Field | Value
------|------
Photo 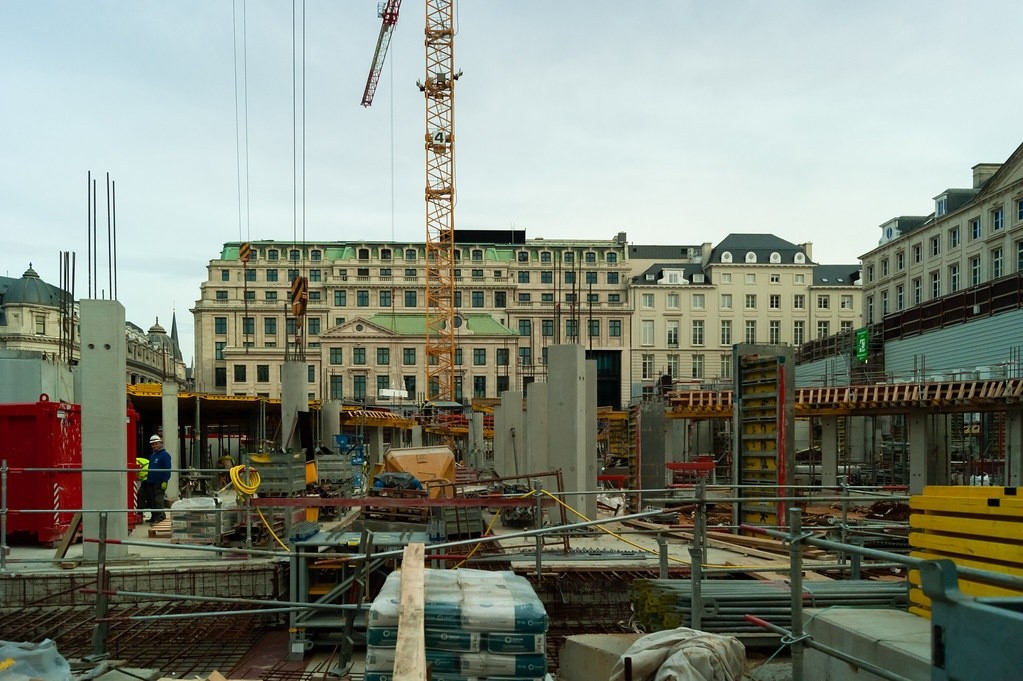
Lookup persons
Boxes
[145,435,172,523]
[218,448,235,489]
[135,457,151,517]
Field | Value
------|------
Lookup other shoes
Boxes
[146,514,166,523]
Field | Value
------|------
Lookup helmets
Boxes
[149,434,163,444]
[223,449,228,453]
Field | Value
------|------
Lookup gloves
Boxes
[161,482,167,489]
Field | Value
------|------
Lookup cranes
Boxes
[359,0,463,511]
